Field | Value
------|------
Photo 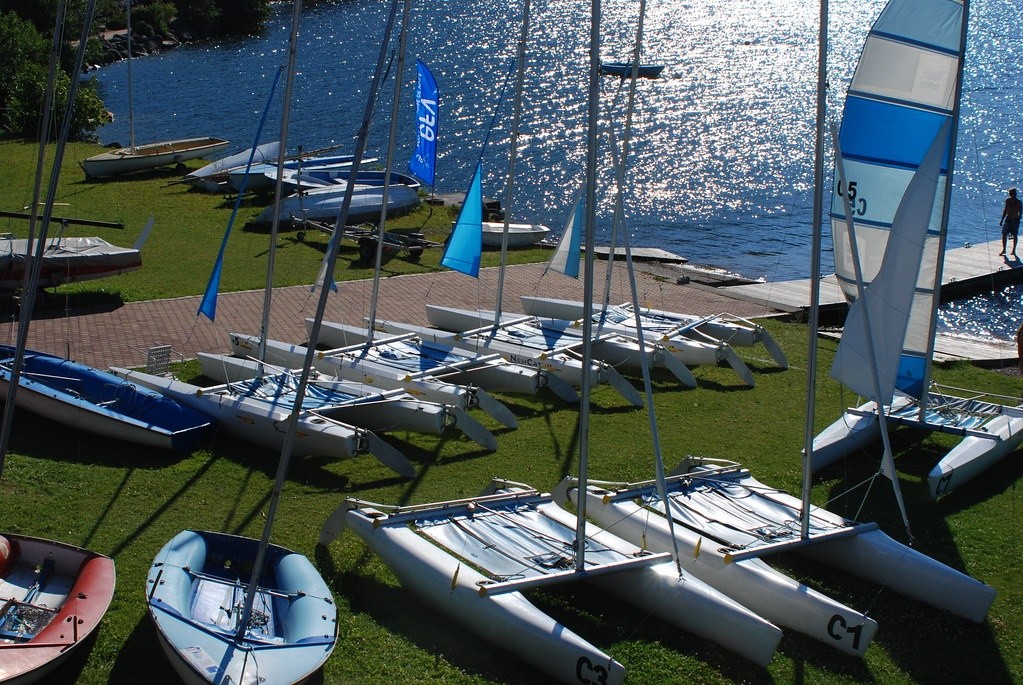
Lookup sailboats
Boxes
[0,0,998,685]
[799,0,1022,505]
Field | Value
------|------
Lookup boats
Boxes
[600,62,664,79]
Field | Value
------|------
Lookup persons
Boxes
[1016,323,1023,372]
[999,187,1023,256]
